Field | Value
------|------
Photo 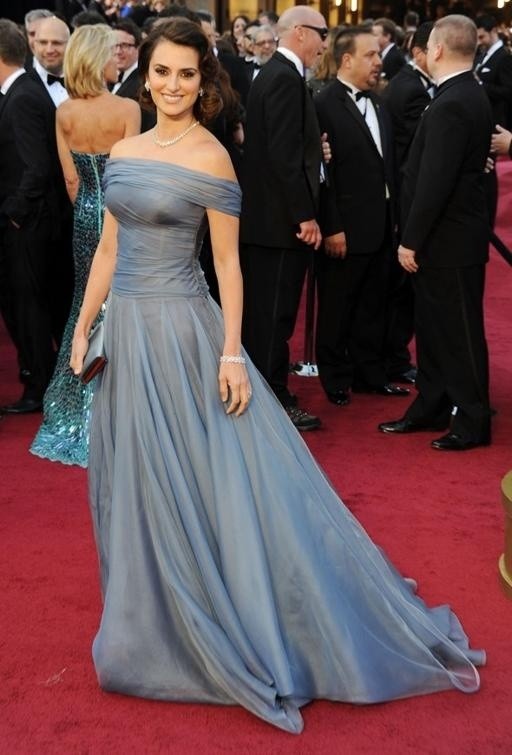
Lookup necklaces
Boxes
[151,121,202,147]
[53,17,486,738]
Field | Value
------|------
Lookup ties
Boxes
[479,51,487,64]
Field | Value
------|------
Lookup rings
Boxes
[247,389,253,399]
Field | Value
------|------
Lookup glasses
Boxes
[243,34,251,39]
[116,42,136,48]
[254,40,275,46]
[304,25,330,41]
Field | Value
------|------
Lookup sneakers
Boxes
[286,405,321,431]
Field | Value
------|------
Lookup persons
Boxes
[29,17,77,107]
[489,125,512,156]
[110,23,144,105]
[75,13,108,28]
[228,15,244,42]
[0,18,74,414]
[233,27,277,99]
[193,12,218,49]
[30,24,141,469]
[78,0,202,23]
[22,10,47,59]
[374,12,495,451]
[231,21,257,63]
[235,7,323,431]
[258,12,276,28]
[312,29,411,407]
[370,18,407,76]
[382,16,443,164]
[472,16,511,121]
[314,21,348,80]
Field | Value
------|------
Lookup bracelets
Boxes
[219,355,246,365]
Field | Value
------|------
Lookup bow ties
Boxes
[419,69,435,91]
[46,73,65,86]
[347,88,374,102]
[252,62,263,71]
[118,71,124,82]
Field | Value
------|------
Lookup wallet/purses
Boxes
[79,321,108,386]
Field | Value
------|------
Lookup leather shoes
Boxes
[329,388,351,406]
[433,432,485,451]
[403,368,418,383]
[0,391,44,414]
[379,384,411,395]
[377,415,423,436]
